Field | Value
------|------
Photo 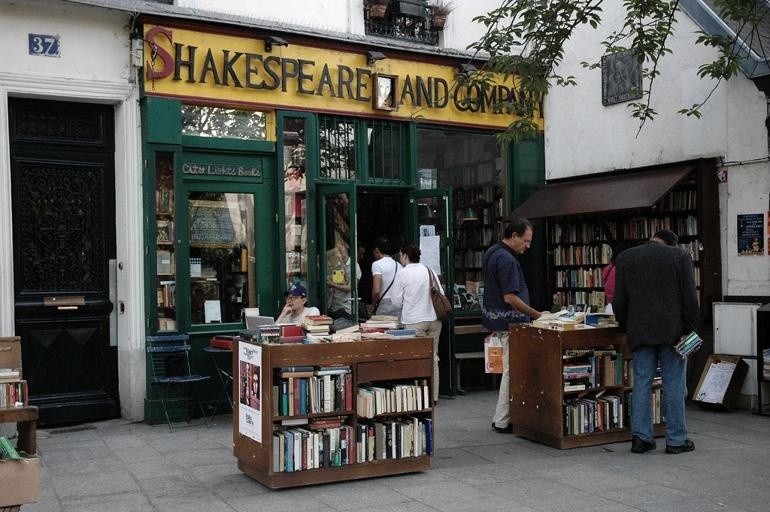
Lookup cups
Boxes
[675,331,703,358]
[561,306,566,312]
[568,305,574,318]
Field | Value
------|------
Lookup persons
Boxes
[602,255,617,308]
[393,245,454,406]
[372,237,405,321]
[613,230,697,454]
[482,218,552,433]
[274,285,319,326]
[326,242,362,334]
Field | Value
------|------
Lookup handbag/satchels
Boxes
[426,265,454,321]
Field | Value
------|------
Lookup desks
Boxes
[202,346,232,420]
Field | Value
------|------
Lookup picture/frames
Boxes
[372,71,399,112]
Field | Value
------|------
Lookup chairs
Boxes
[145,333,211,434]
[452,315,505,395]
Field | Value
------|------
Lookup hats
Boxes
[284,284,307,297]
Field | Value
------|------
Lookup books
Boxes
[156,220,174,244]
[555,270,605,287]
[156,186,174,213]
[656,388,661,425]
[357,418,434,463]
[652,394,656,424]
[274,367,353,415]
[441,144,497,167]
[655,188,698,209]
[454,223,503,245]
[273,416,353,472]
[454,208,498,223]
[158,281,175,308]
[554,244,614,263]
[529,310,618,331]
[553,215,697,242]
[453,271,485,308]
[674,243,701,260]
[451,185,500,206]
[246,308,273,332]
[654,367,665,390]
[556,291,606,308]
[562,348,634,388]
[190,261,218,279]
[0,383,22,405]
[159,313,174,331]
[188,197,246,242]
[356,380,430,418]
[241,315,417,345]
[439,163,500,183]
[565,394,628,435]
[156,250,176,275]
[454,246,485,268]
[762,349,770,378]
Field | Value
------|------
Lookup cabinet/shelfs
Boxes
[503,167,722,344]
[380,127,503,291]
[156,156,307,331]
[507,324,677,450]
[229,336,433,490]
[0,334,40,512]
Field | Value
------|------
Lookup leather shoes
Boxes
[631,435,657,454]
[665,439,695,454]
[492,422,495,430]
[495,422,513,433]
